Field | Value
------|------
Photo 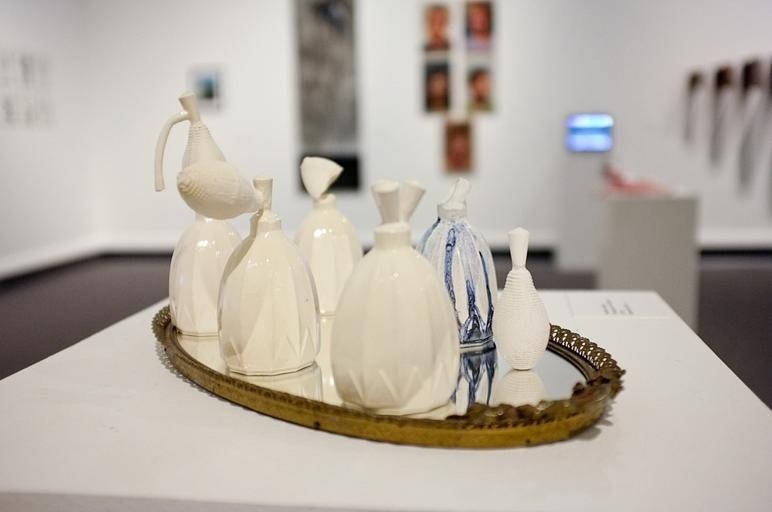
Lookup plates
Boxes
[152,306,627,450]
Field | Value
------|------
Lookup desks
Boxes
[0,288,770,510]
